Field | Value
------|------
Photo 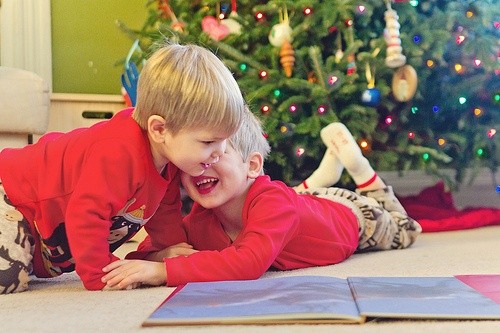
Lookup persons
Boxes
[105,100,424,288]
[0,44,246,295]
